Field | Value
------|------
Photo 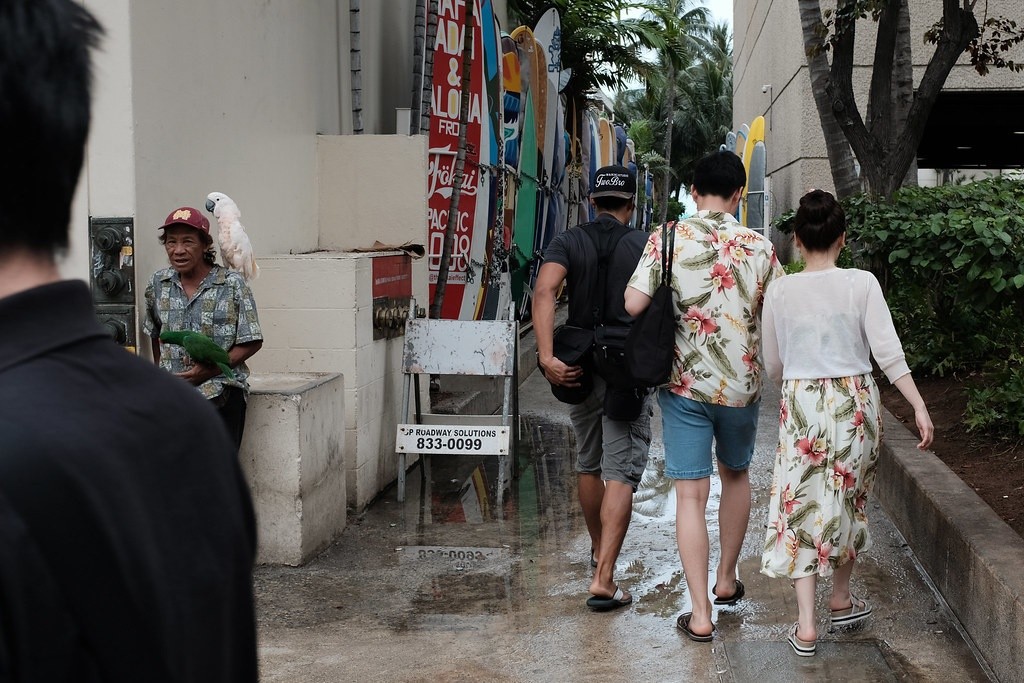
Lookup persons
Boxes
[0,0,265,683]
[762,190,933,656]
[623,151,788,642]
[141,207,265,456]
[531,163,650,610]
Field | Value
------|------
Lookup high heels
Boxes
[786,620,816,657]
[830,591,873,626]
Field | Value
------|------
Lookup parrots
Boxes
[204,192,260,283]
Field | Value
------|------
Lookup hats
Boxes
[590,164,636,200]
[158,207,210,235]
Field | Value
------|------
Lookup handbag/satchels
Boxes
[592,317,633,383]
[535,324,597,405]
[601,377,645,424]
[621,219,679,386]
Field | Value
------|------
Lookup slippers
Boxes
[712,579,745,605]
[591,547,599,567]
[677,612,716,642]
[586,586,633,608]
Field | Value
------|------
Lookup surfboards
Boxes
[425,0,655,321]
[718,116,767,236]
